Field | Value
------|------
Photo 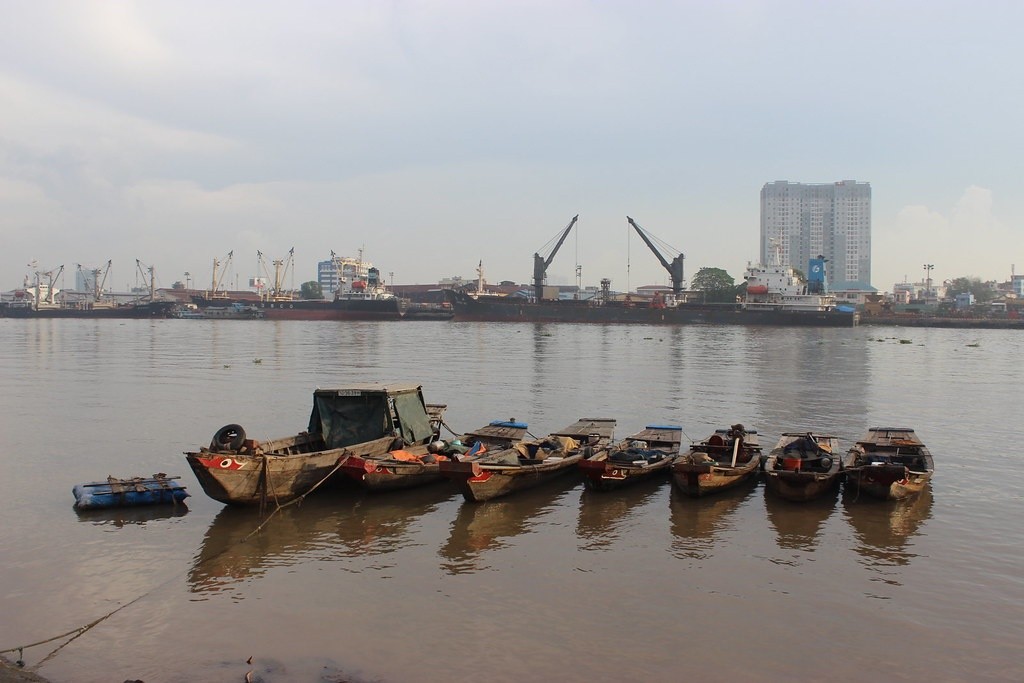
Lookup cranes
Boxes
[79,261,117,315]
[256,249,296,304]
[135,260,182,317]
[210,252,236,304]
[34,264,65,307]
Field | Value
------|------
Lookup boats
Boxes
[183,389,445,500]
[331,248,455,317]
[764,435,841,502]
[445,421,616,500]
[448,260,513,304]
[341,415,525,496]
[844,428,932,505]
[671,428,758,496]
[573,427,675,490]
[743,238,863,327]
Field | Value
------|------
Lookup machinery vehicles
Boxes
[530,215,583,309]
[627,213,686,307]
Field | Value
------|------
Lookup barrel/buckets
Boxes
[783,458,801,471]
